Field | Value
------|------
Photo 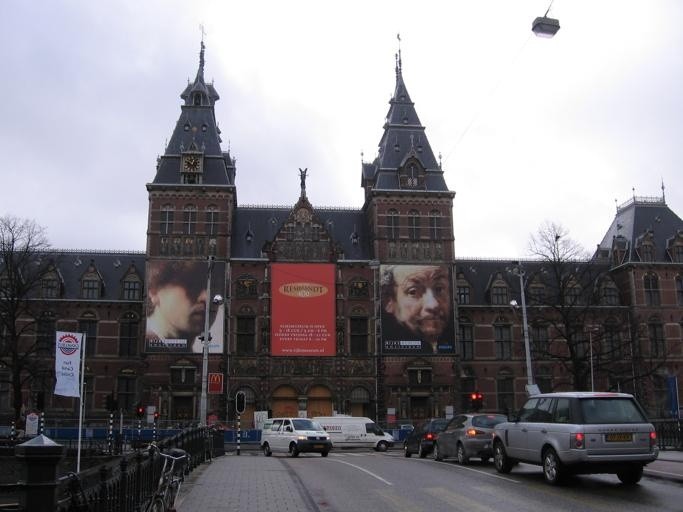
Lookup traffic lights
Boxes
[101,392,111,410]
[477,393,483,409]
[154,412,160,423]
[236,391,247,415]
[138,407,145,417]
[471,392,477,408]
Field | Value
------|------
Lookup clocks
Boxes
[178,151,204,175]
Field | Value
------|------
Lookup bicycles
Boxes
[198,424,223,463]
[138,440,190,512]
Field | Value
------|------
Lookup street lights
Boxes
[509,270,534,385]
[589,326,599,393]
[198,255,224,430]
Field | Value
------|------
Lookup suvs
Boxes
[492,390,661,487]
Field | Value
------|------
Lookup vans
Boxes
[261,417,333,458]
[313,416,395,452]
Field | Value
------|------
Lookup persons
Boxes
[380,264,455,354]
[146,259,224,352]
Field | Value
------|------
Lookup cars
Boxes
[404,416,452,459]
[433,412,509,465]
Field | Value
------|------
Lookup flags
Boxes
[54,331,83,398]
